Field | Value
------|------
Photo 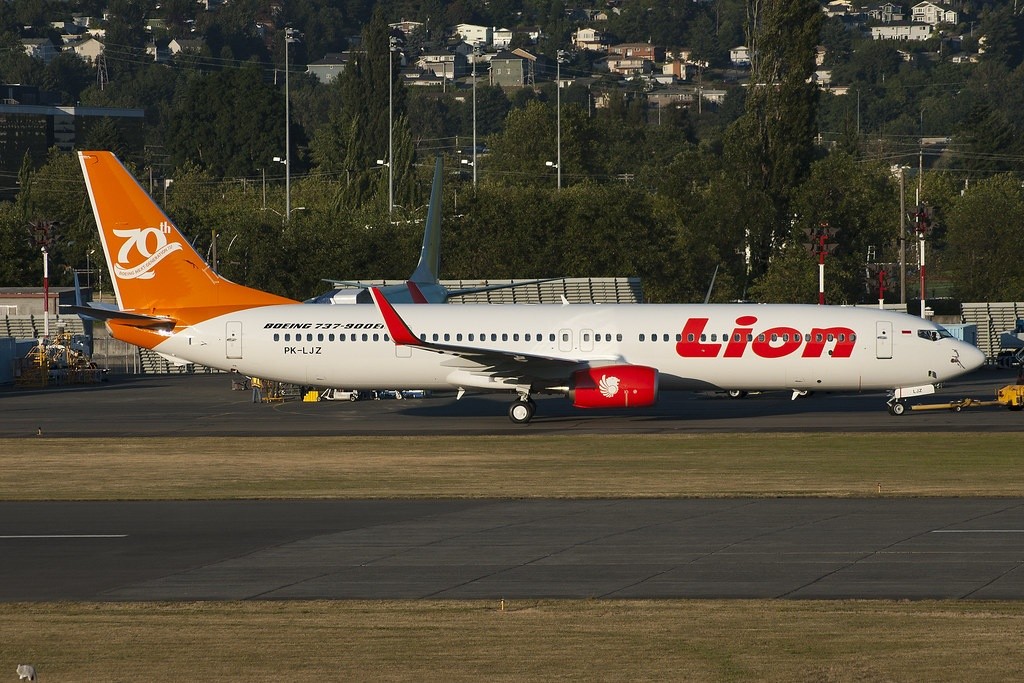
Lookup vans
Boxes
[322,385,372,401]
[384,385,430,398]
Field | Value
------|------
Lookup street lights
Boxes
[549,48,571,194]
[385,35,407,217]
[282,25,307,223]
[471,41,487,204]
[258,203,306,246]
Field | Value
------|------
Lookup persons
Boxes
[250,376,263,403]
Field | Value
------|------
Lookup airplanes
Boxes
[73,149,987,427]
[303,153,575,305]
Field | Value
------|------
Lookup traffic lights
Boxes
[906,207,919,237]
